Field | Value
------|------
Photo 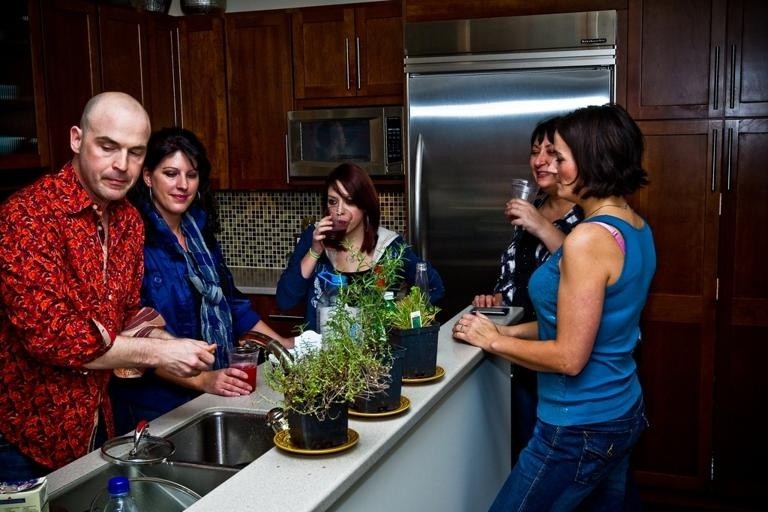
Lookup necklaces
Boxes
[584,203,631,218]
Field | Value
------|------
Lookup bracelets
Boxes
[310,247,319,261]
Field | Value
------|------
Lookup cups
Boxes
[226,344,261,394]
[319,206,354,249]
[503,177,539,226]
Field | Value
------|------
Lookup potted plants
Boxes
[384,289,439,379]
[264,321,391,449]
[325,237,413,412]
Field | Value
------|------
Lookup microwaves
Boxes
[285,105,405,181]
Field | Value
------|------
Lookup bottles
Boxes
[104,476,138,512]
[384,292,397,312]
[413,262,429,301]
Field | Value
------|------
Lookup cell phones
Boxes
[471,306,509,316]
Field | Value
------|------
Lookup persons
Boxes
[452,105,657,512]
[0,92,216,485]
[471,116,586,472]
[107,126,295,439]
[274,164,445,333]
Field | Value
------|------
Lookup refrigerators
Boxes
[402,9,621,324]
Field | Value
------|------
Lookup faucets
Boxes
[97,417,181,490]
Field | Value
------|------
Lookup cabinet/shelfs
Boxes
[0,0,230,194]
[225,8,294,191]
[287,0,405,189]
[626,0,768,512]
[28,306,522,512]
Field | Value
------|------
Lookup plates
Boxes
[274,427,359,456]
[402,366,447,386]
[348,393,410,419]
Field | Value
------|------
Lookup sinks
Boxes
[42,363,293,508]
